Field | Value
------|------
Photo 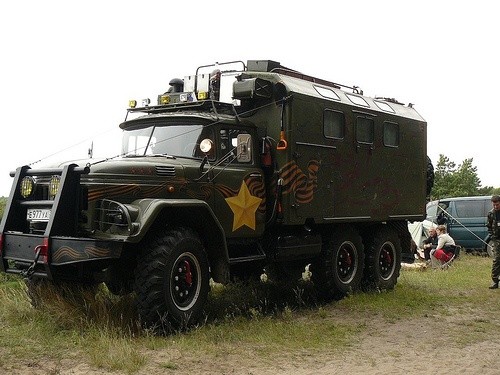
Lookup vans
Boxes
[425,195,494,258]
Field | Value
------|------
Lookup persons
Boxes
[430,225,455,261]
[486,195,500,289]
[423,227,438,262]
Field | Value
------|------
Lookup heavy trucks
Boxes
[1,58,434,338]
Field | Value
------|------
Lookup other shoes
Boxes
[489,283,498,289]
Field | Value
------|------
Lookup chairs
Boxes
[440,246,461,270]
